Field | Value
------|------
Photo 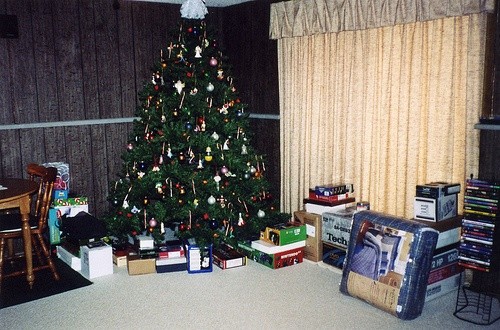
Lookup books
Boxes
[457,179,500,273]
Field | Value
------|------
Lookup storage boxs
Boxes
[42,162,460,301]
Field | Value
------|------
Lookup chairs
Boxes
[0,164,60,284]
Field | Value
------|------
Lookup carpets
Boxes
[0,212,92,311]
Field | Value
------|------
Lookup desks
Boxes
[0,178,38,289]
